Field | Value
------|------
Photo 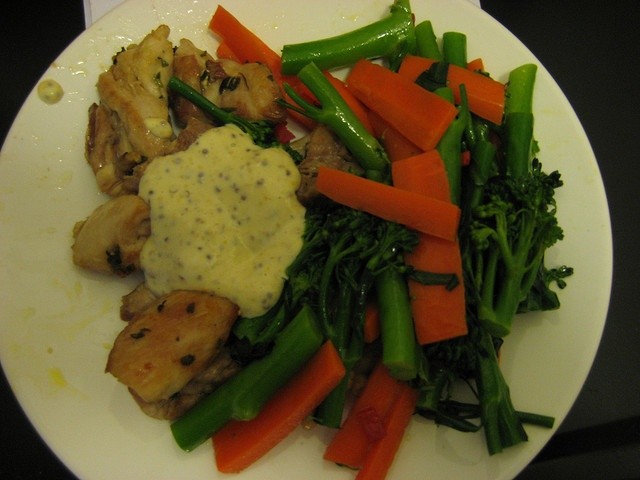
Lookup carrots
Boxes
[212,0,506,479]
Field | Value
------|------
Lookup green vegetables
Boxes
[165,1,574,454]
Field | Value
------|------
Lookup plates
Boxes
[0,0,614,480]
